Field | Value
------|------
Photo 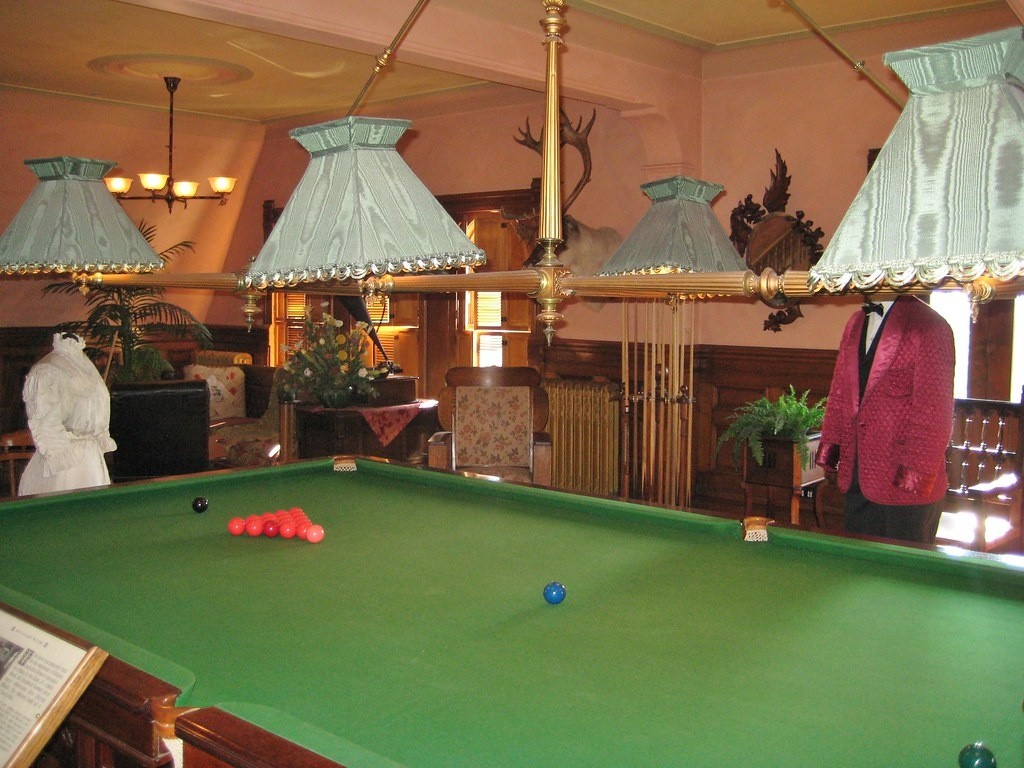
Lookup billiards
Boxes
[227,518,245,536]
[542,581,568,604]
[246,506,311,540]
[192,497,209,513]
[307,525,325,543]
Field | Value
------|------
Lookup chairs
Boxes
[2,431,33,495]
[427,365,552,487]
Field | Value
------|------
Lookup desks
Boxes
[0,451,1024,768]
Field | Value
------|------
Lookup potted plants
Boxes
[714,385,828,472]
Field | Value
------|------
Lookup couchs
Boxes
[108,366,279,482]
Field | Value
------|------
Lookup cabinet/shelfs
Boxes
[294,397,440,462]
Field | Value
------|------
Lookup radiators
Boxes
[538,381,626,495]
[197,349,253,365]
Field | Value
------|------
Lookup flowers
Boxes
[270,301,390,399]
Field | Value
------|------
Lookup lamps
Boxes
[597,177,752,273]
[240,115,491,292]
[0,155,165,274]
[805,27,1024,288]
[104,77,238,215]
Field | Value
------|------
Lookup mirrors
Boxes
[749,213,815,308]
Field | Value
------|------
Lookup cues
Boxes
[618,298,697,510]
[102,328,118,384]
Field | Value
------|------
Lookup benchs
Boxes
[812,398,1024,555]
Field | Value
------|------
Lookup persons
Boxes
[815,294,956,542]
[18,332,117,496]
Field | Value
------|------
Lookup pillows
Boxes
[183,364,247,421]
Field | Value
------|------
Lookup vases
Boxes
[319,388,352,407]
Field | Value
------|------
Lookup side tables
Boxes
[738,432,825,524]
